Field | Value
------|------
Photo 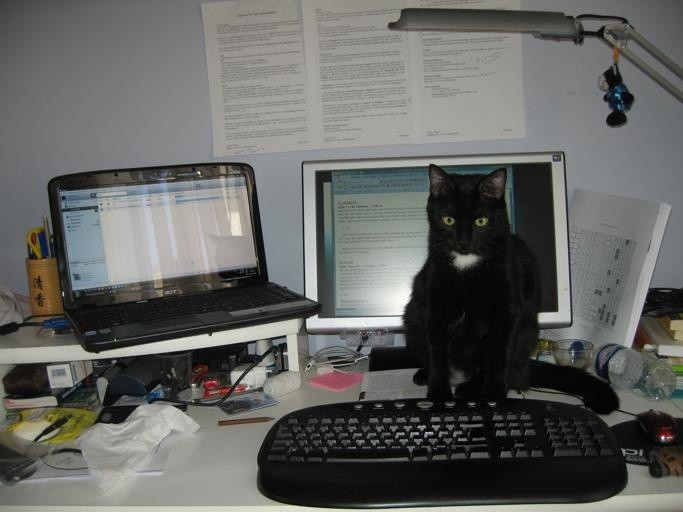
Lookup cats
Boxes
[401,163,620,416]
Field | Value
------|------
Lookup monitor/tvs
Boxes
[302,151,573,371]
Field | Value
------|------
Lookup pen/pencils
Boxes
[219,417,274,425]
[27,216,55,259]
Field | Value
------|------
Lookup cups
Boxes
[154,351,194,402]
[23,257,64,322]
[552,337,595,371]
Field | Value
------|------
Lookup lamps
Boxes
[384,8,681,106]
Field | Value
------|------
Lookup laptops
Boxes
[48,162,322,352]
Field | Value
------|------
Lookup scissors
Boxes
[27,226,45,259]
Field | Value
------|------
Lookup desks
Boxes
[2,353,683,510]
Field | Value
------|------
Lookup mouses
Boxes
[636,409,680,444]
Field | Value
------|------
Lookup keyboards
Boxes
[257,398,628,508]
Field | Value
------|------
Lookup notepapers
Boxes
[309,372,364,393]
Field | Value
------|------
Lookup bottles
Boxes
[263,370,303,397]
[590,342,676,399]
[254,340,276,366]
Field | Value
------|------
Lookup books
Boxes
[633,312,683,400]
